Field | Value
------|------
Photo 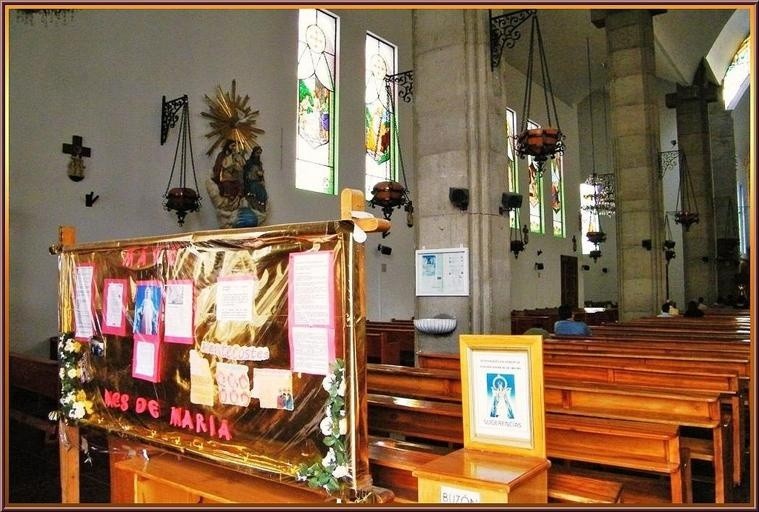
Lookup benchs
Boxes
[10,299,751,505]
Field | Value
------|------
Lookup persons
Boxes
[553,305,592,336]
[658,297,724,318]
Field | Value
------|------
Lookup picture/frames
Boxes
[414,248,470,296]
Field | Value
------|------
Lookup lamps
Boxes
[718,198,740,257]
[587,183,607,246]
[588,242,603,262]
[509,208,525,259]
[663,214,675,249]
[673,147,700,230]
[366,75,409,218]
[160,95,202,225]
[515,11,565,162]
